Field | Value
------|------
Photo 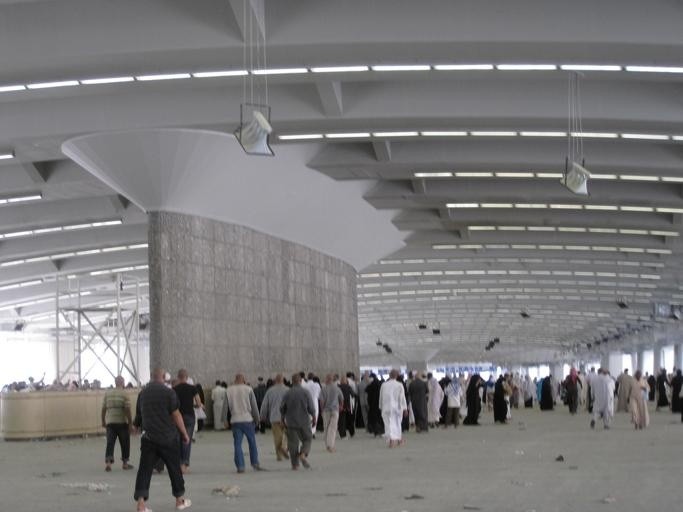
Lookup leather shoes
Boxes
[237,470,244,473]
[252,464,262,470]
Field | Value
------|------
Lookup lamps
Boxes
[230,0,280,158]
[555,70,597,199]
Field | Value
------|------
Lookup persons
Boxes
[132,364,191,512]
[485,365,683,431]
[2,371,100,391]
[163,368,484,475]
[100,374,134,472]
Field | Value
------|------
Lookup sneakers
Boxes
[176,499,191,510]
[276,447,310,470]
[144,507,153,512]
[106,466,111,472]
[123,464,134,469]
